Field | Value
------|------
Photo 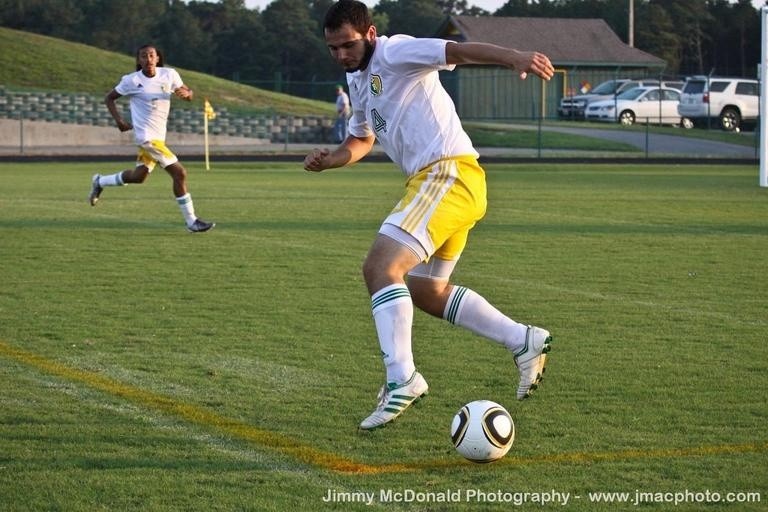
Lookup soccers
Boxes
[451,400,515,463]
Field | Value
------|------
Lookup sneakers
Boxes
[360,370,429,430]
[185,219,215,234]
[514,325,552,403]
[89,174,103,207]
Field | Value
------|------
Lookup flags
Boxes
[205,101,218,120]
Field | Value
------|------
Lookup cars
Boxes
[556,74,760,130]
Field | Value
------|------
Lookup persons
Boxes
[88,44,214,234]
[334,85,352,144]
[301,0,555,429]
